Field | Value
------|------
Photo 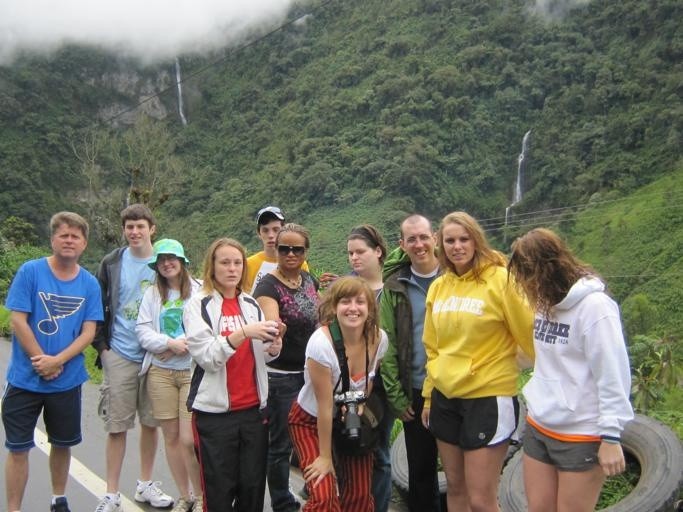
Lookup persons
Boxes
[422,213,535,510]
[182,239,282,511]
[377,214,447,511]
[504,227,636,511]
[91,204,175,512]
[344,227,393,308]
[1,211,105,511]
[135,238,204,512]
[288,275,389,511]
[240,206,312,297]
[250,224,327,511]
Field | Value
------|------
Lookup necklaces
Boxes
[275,265,304,289]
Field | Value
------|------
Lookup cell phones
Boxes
[263,321,277,349]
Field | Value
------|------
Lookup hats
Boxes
[148,239,189,270]
[256,206,284,224]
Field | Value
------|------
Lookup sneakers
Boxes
[51,496,70,512]
[135,480,174,507]
[171,495,203,512]
[95,493,123,512]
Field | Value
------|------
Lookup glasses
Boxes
[278,245,305,256]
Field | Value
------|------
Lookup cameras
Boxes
[333,390,368,440]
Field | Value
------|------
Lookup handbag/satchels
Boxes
[334,414,374,455]
